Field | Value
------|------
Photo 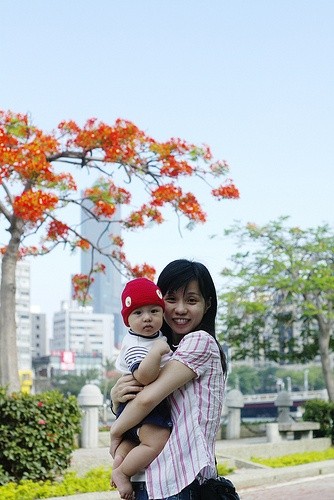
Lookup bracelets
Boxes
[110,399,116,416]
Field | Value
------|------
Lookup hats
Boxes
[120,277,166,328]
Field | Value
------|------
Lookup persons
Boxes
[104,277,174,499]
[109,259,226,500]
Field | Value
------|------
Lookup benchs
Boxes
[259,421,321,440]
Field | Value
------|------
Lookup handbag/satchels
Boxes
[191,477,240,500]
